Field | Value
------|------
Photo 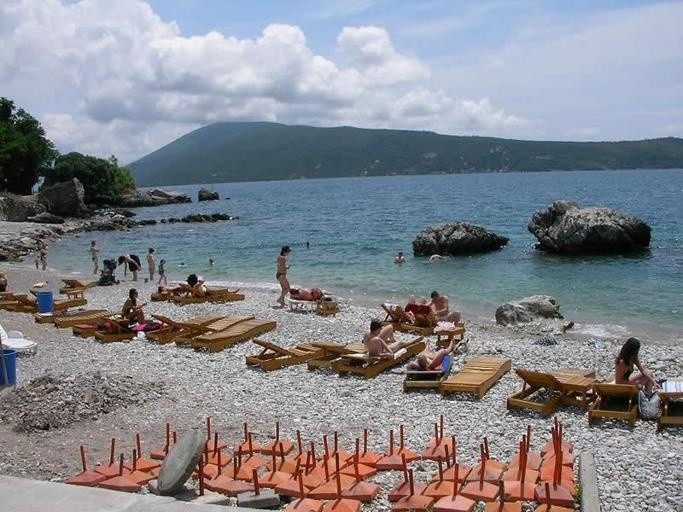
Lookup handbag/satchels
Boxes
[636,389,664,420]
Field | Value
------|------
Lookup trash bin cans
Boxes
[1,349,18,386]
[39,292,53,312]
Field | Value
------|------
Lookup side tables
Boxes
[561,377,599,409]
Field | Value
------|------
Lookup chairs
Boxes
[289,295,336,317]
[72,313,277,347]
[658,377,682,431]
[248,337,425,378]
[507,368,596,419]
[376,301,466,350]
[402,349,454,391]
[589,376,638,430]
[151,285,245,305]
[3,275,118,329]
[440,359,511,400]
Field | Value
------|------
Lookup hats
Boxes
[281,245,291,252]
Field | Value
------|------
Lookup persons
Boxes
[615,337,662,397]
[363,290,460,370]
[394,252,406,264]
[276,245,292,305]
[36,256,39,269]
[90,241,214,324]
[42,257,47,270]
[289,288,332,301]
